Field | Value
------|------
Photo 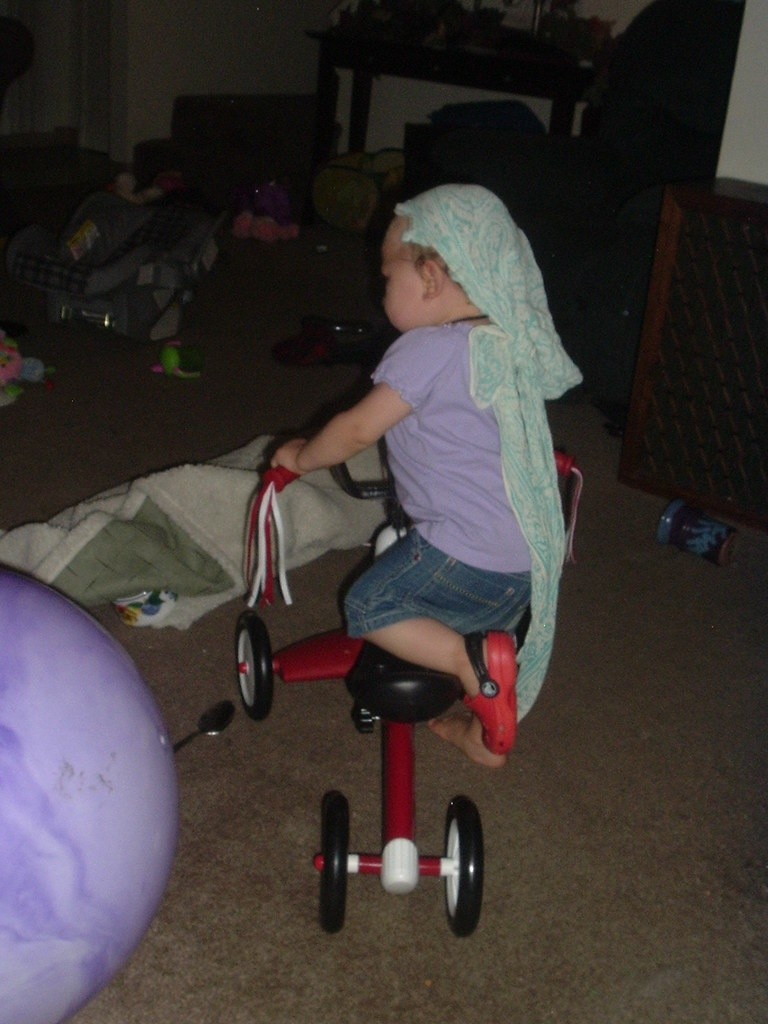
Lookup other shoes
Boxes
[272,314,354,367]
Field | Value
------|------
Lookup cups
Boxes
[655,497,739,567]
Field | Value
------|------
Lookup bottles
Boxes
[303,315,389,347]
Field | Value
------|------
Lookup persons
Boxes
[271,183,584,769]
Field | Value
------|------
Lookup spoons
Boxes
[171,701,236,754]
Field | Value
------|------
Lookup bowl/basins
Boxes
[113,589,178,630]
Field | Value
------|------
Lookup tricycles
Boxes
[237,447,580,938]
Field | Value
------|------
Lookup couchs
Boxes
[133,92,342,221]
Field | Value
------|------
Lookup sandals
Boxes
[463,630,518,756]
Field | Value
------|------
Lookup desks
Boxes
[301,24,599,224]
